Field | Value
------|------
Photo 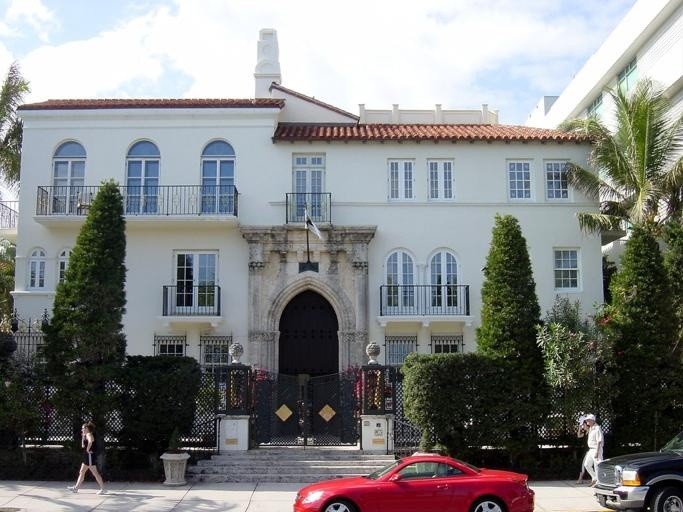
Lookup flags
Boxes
[305,208,324,242]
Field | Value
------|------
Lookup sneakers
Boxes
[65,485,78,493]
[586,478,598,488]
[95,487,108,495]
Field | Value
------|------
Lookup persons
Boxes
[66,421,108,495]
[583,414,604,487]
[575,419,590,484]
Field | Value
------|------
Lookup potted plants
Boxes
[158,427,190,486]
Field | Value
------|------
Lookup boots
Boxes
[575,472,585,484]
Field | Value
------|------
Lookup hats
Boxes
[579,415,587,425]
[583,413,596,421]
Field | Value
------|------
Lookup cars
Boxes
[290,456,535,512]
[537,413,575,442]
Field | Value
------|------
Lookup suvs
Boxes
[595,432,682,511]
